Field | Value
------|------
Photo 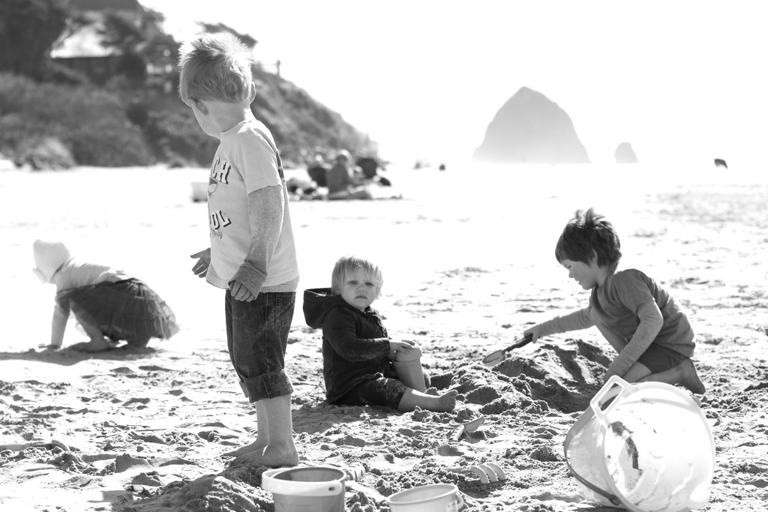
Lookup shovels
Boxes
[482,336,541,364]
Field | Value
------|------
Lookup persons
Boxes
[175,29,300,473]
[28,226,181,354]
[284,147,394,201]
[517,207,710,404]
[301,254,459,416]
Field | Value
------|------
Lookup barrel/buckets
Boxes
[389,483,464,512]
[261,465,346,511]
[564,374,717,512]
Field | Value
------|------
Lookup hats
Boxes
[33,240,69,281]
